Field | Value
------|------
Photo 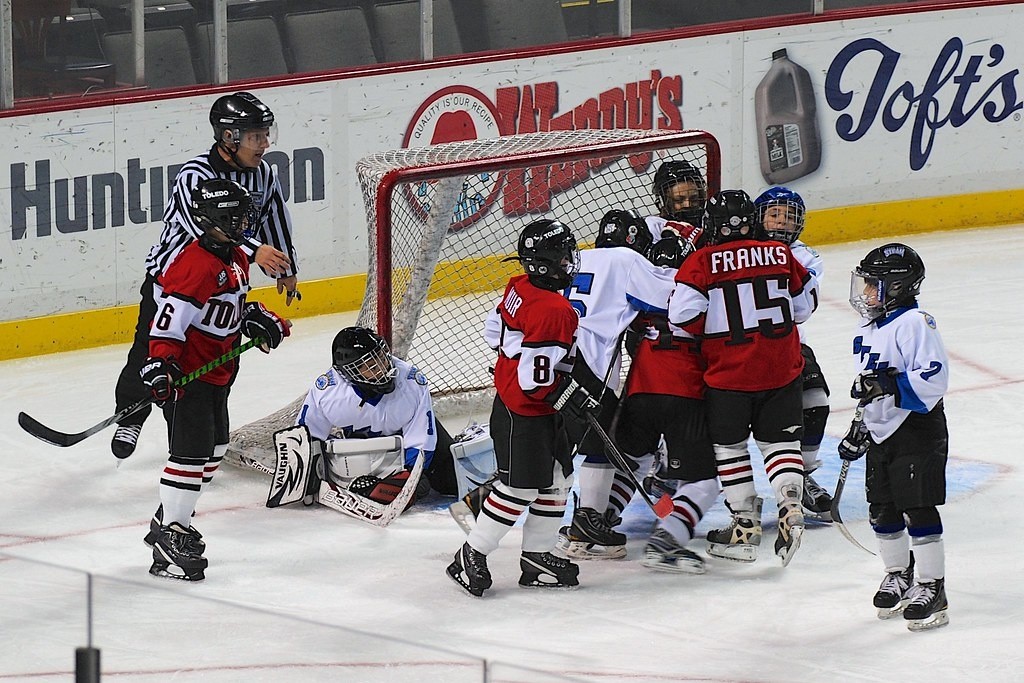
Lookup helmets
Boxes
[648,236,695,268]
[210,92,274,130]
[848,242,925,319]
[518,219,582,291]
[192,179,259,248]
[753,186,806,246]
[652,158,706,227]
[332,326,399,393]
[701,190,759,246]
[595,208,653,258]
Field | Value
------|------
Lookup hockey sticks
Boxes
[831,379,877,557]
[224,448,425,528]
[570,330,627,459]
[19,318,294,447]
[584,409,675,518]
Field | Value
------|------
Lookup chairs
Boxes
[103,0,565,88]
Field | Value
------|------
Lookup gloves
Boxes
[838,419,872,462]
[544,375,603,425]
[850,367,896,408]
[239,301,290,353]
[139,357,190,409]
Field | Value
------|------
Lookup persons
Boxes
[270,325,495,528]
[447,159,833,596]
[109,92,302,468]
[137,178,290,582]
[839,243,949,629]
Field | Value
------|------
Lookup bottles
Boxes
[754,48,822,185]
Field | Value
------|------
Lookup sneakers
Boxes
[567,491,628,560]
[773,485,806,567]
[873,550,915,619]
[448,485,493,536]
[642,458,680,504]
[706,496,764,561]
[110,424,143,469]
[149,508,207,581]
[445,541,492,598]
[555,508,623,552]
[518,551,580,591]
[903,576,949,631]
[143,503,205,556]
[801,468,836,527]
[639,516,706,574]
